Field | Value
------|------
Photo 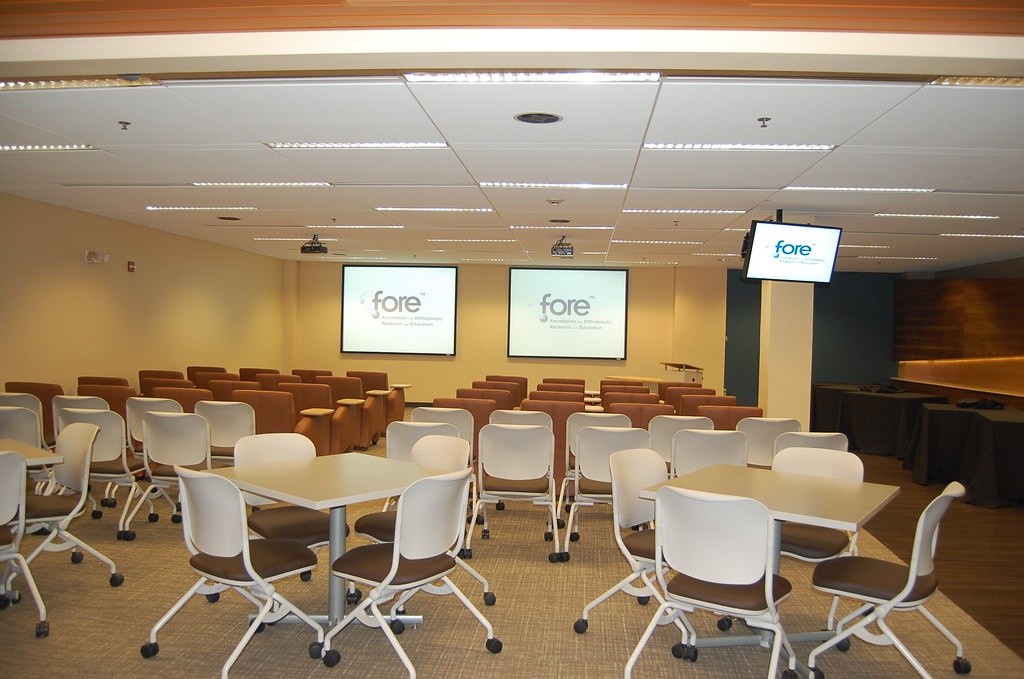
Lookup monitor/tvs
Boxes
[740,219,842,285]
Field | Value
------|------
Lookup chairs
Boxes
[0,367,970,679]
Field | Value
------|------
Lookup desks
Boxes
[0,437,64,611]
[200,450,458,659]
[836,392,947,458]
[902,396,1024,509]
[812,382,883,432]
[639,464,900,679]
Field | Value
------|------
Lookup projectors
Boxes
[301,245,328,253]
[550,246,574,258]
